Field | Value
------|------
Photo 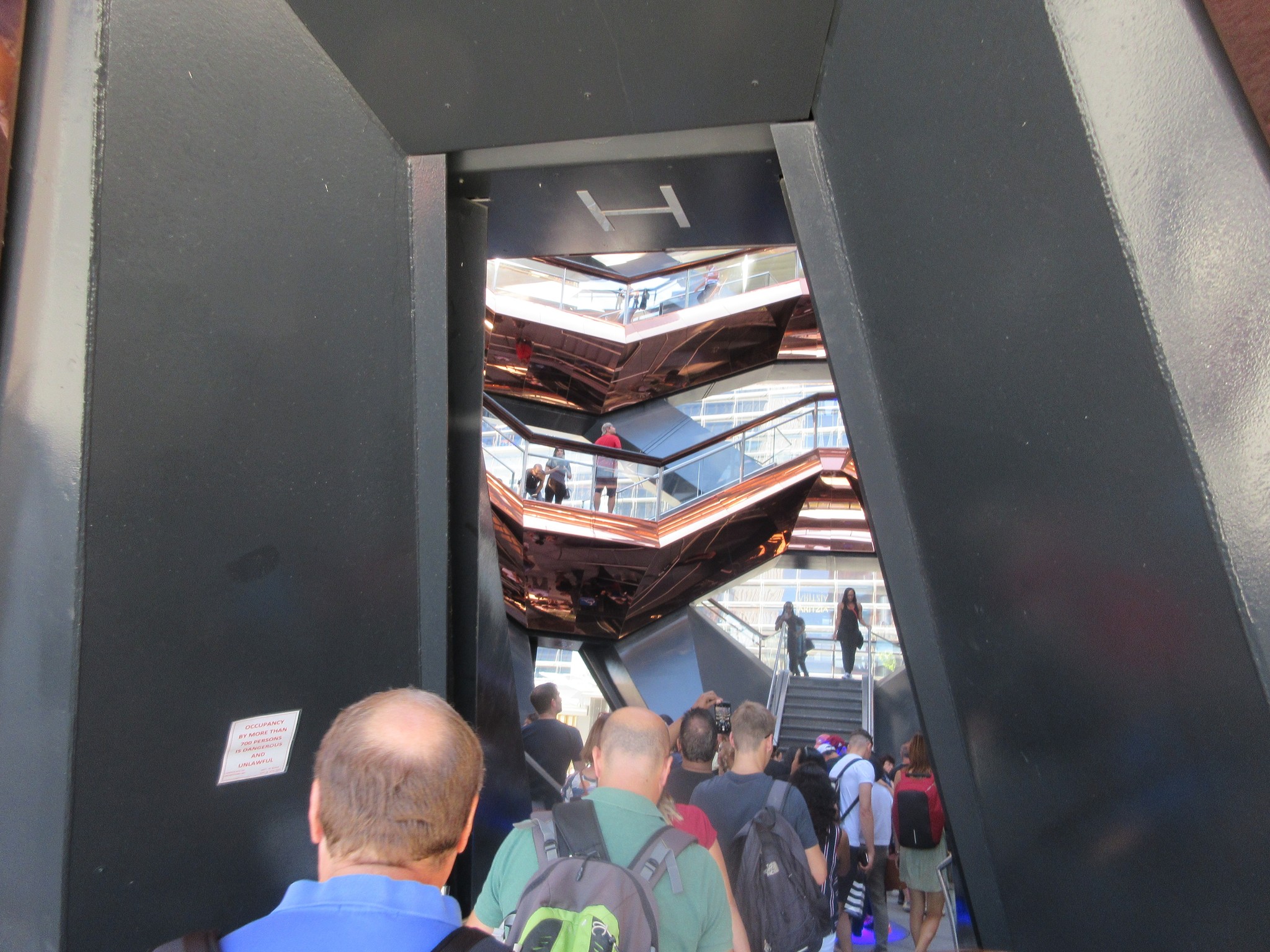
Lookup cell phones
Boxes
[861,852,868,867]
[714,701,731,734]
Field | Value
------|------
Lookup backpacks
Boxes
[733,781,832,952]
[891,769,944,848]
[499,798,698,952]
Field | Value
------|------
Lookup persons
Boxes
[521,682,948,952]
[544,447,572,505]
[629,370,680,398]
[518,464,546,501]
[616,286,650,310]
[154,690,514,952]
[832,588,872,679]
[593,423,622,514]
[695,263,719,303]
[516,334,532,367]
[463,705,733,952]
[775,602,809,678]
[523,532,640,612]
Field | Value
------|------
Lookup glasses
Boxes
[785,606,791,609]
[802,743,809,756]
[597,711,608,719]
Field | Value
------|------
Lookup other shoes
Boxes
[795,673,800,677]
[842,672,851,680]
[902,900,926,914]
[804,672,809,677]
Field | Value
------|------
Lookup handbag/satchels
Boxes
[806,637,815,652]
[857,629,863,649]
[836,628,844,642]
[843,875,867,918]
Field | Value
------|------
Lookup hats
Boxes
[818,744,836,755]
[830,735,845,749]
[813,734,829,748]
